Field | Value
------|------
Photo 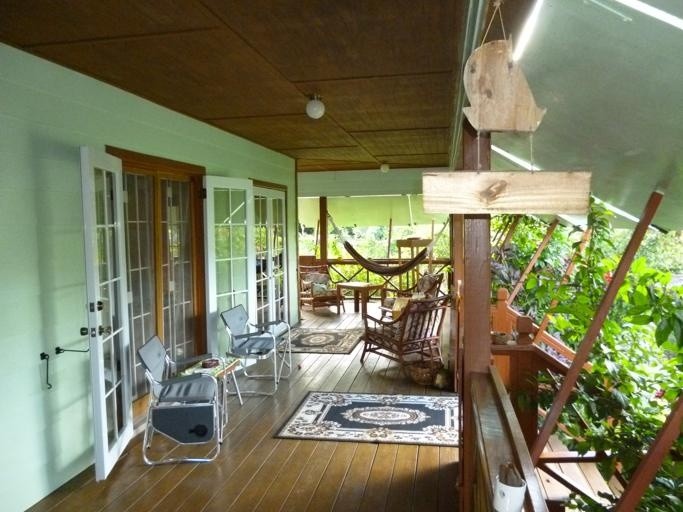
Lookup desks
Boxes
[336,281,386,315]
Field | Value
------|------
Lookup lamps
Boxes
[379,159,392,175]
[303,91,329,121]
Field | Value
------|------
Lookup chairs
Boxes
[359,294,451,377]
[219,304,292,396]
[379,271,445,324]
[298,264,347,315]
[136,335,228,466]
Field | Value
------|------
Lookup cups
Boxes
[492,474,526,512]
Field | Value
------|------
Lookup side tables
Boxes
[179,356,244,407]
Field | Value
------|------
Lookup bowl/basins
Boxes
[490,333,508,345]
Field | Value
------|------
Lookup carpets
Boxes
[274,327,370,355]
[272,391,461,448]
